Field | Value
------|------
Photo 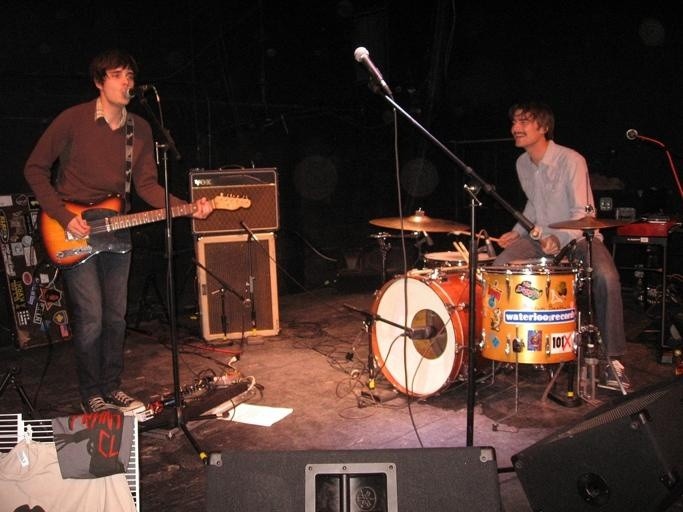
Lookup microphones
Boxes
[249,275,256,327]
[626,128,638,140]
[125,84,153,99]
[240,220,259,242]
[353,46,392,97]
[549,238,576,266]
[407,326,435,340]
[484,231,496,257]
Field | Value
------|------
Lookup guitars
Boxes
[37,191,252,270]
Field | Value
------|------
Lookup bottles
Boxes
[584,343,598,378]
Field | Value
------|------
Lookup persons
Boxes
[24,48,217,414]
[475,101,631,390]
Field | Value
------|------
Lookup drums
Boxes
[424,250,497,274]
[480,256,582,370]
[369,269,502,398]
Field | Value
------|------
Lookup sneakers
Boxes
[108,390,142,412]
[601,360,629,389]
[80,397,108,413]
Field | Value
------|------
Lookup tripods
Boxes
[139,98,217,466]
[539,236,628,402]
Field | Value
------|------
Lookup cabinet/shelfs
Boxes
[612,238,680,347]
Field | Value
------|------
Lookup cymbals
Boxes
[547,217,630,230]
[368,217,472,233]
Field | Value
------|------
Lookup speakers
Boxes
[207,446,502,511]
[194,231,280,341]
[510,376,683,512]
[188,167,281,234]
